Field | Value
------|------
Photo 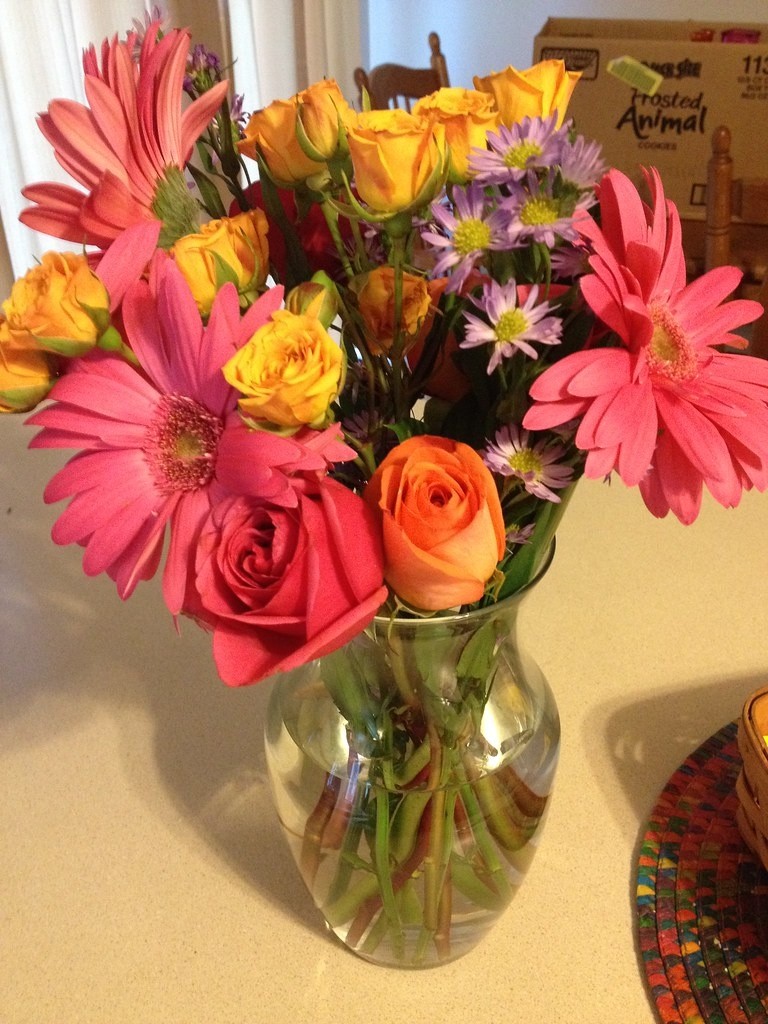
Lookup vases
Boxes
[266,536,562,972]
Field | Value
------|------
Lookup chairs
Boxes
[350,31,449,115]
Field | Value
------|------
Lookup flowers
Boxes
[0,8,768,674]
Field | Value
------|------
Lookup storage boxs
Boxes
[525,16,768,227]
